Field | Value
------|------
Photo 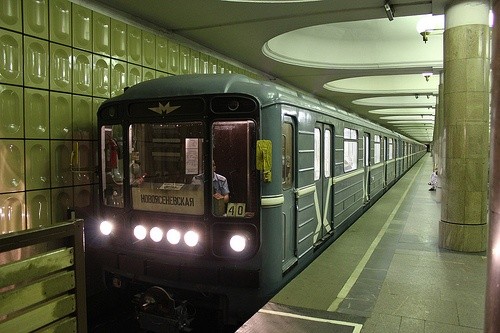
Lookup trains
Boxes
[97,73,428,333]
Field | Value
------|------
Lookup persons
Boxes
[427,164,438,191]
[192,159,231,203]
[106,146,145,209]
